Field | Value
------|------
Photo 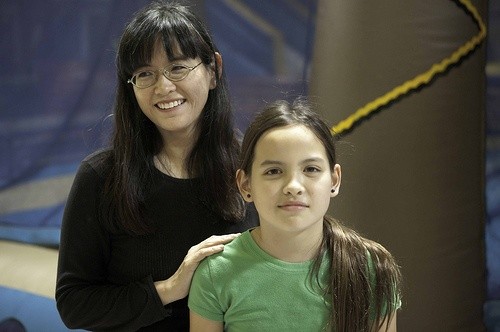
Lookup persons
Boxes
[187,98,402,332]
[54,1,261,331]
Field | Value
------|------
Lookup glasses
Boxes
[127,60,204,89]
